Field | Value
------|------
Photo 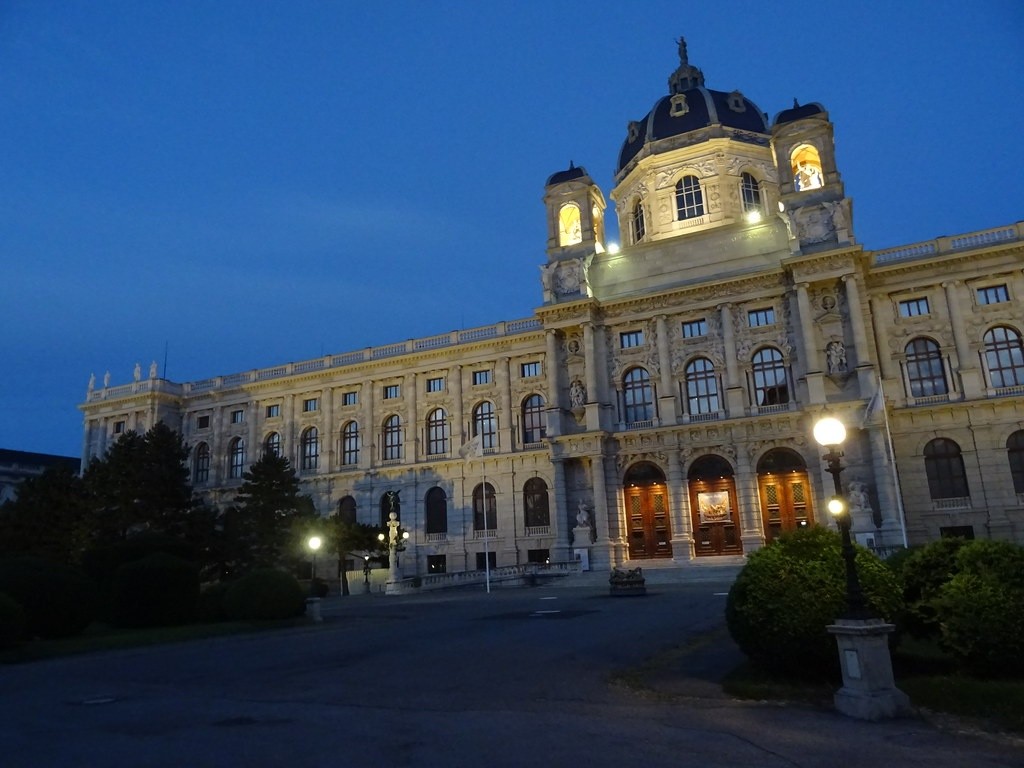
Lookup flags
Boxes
[457,434,483,464]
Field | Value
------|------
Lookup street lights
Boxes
[810,414,912,726]
[305,535,322,602]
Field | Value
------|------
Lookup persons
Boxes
[88,373,96,391]
[673,35,689,61]
[149,360,157,378]
[103,371,112,387]
[569,383,584,406]
[134,364,142,381]
[826,342,848,373]
[575,498,593,527]
